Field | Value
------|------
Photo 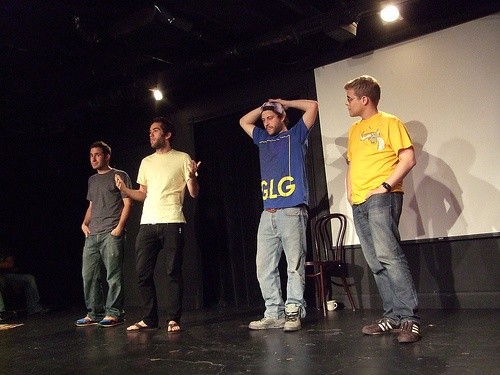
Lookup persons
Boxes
[344,77,422,343]
[74,141,133,327]
[239,98,318,332]
[115,117,201,331]
[0,252,19,322]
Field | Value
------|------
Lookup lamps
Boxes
[339,17,359,38]
[376,5,404,26]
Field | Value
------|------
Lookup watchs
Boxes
[382,182,392,191]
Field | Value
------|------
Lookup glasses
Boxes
[347,97,356,103]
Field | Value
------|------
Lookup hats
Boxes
[260,102,289,122]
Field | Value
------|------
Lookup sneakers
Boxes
[98,316,126,328]
[398,320,422,342]
[74,315,99,326]
[362,317,401,335]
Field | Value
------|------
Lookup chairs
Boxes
[304,215,358,317]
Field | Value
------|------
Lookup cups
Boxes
[326,299,338,311]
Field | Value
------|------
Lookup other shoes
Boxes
[283,304,302,331]
[26,308,48,320]
[248,316,286,329]
[0,312,11,321]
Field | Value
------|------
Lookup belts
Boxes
[266,204,303,213]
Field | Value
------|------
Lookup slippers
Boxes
[126,320,158,331]
[167,320,181,334]
